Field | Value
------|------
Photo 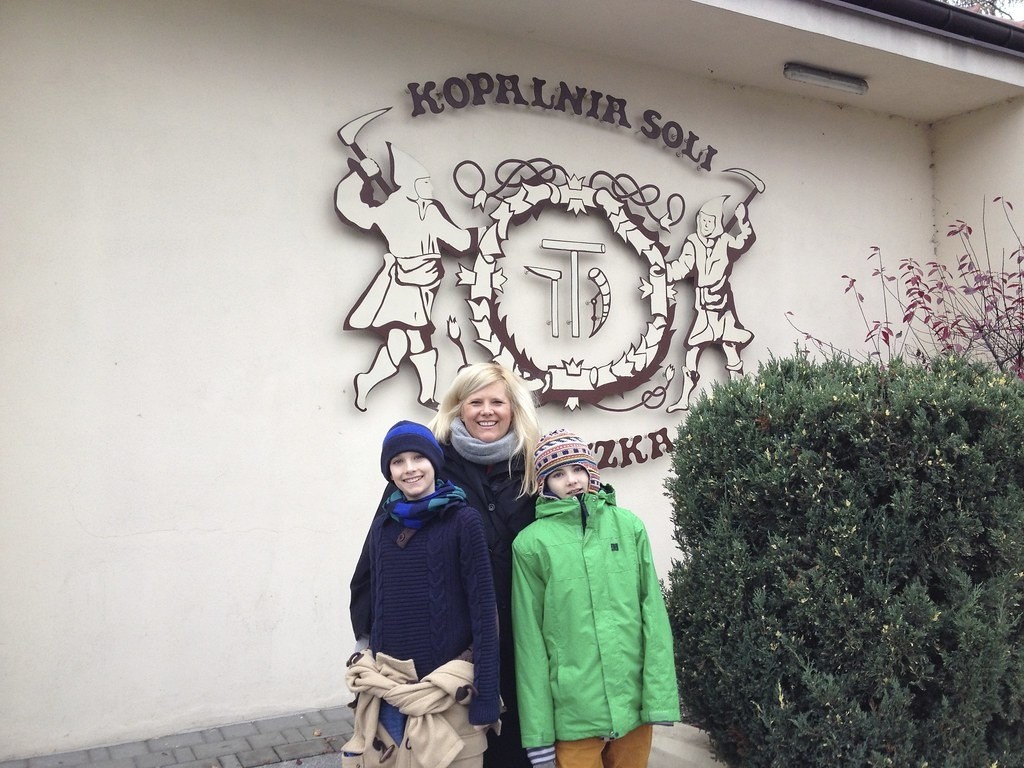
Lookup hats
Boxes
[380,419,444,483]
[535,426,600,499]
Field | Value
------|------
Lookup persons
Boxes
[368,420,505,768]
[350,362,542,768]
[511,430,680,768]
